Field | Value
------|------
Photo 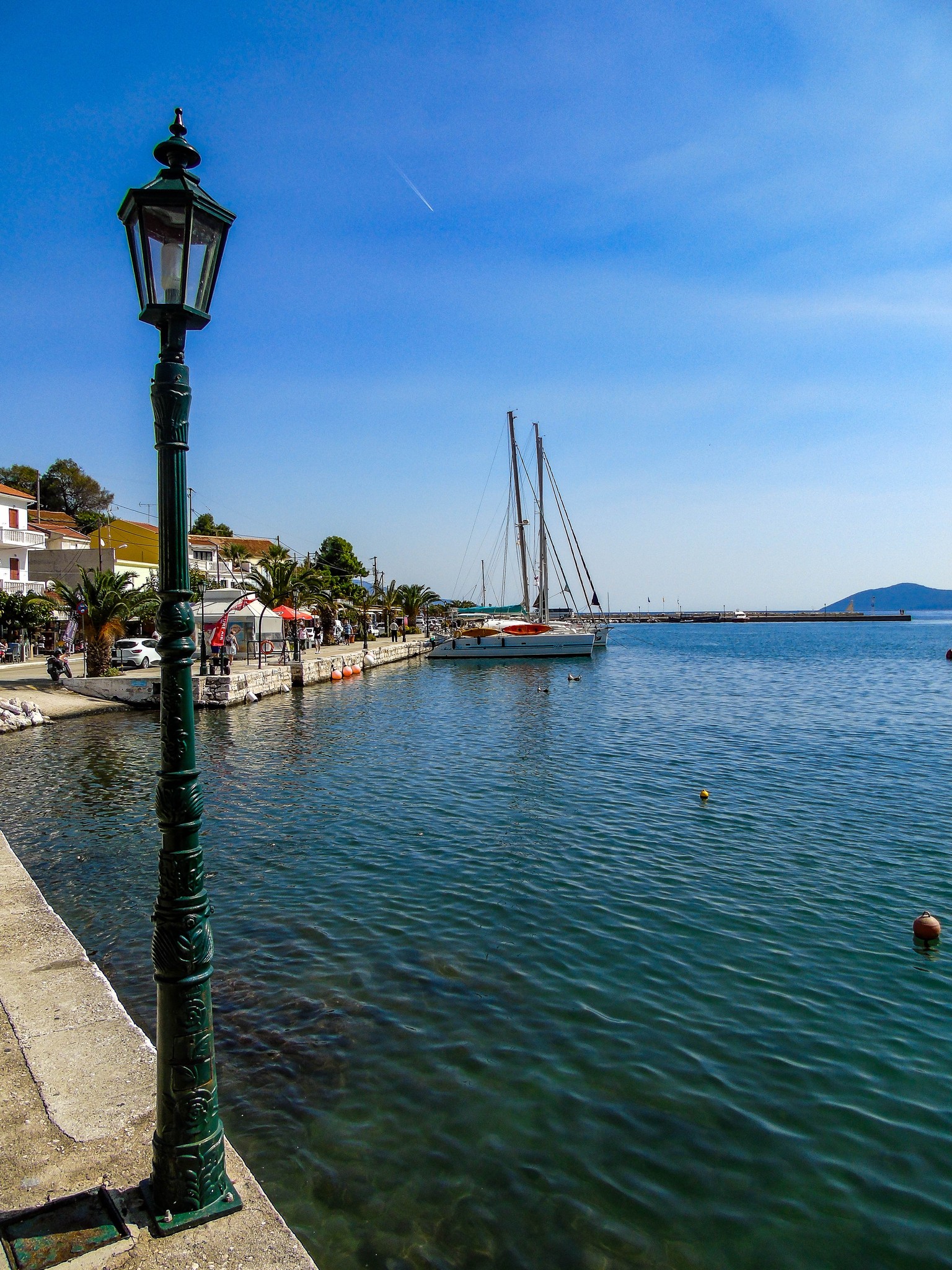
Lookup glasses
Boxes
[301,627,304,628]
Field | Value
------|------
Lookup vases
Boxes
[347,635,355,643]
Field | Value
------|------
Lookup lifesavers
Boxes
[261,640,274,655]
[433,625,440,632]
[560,615,564,619]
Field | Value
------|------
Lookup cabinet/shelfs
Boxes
[38,630,59,654]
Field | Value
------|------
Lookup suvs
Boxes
[377,623,385,636]
[334,619,344,630]
[111,638,161,669]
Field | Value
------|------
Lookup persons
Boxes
[900,609,904,615]
[211,631,239,666]
[344,620,354,646]
[284,618,322,655]
[0,635,29,664]
[390,620,399,642]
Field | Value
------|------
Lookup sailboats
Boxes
[424,406,614,659]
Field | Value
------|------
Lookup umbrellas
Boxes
[271,605,312,641]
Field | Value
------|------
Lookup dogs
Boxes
[901,614,903,615]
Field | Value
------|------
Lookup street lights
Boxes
[362,593,368,649]
[99,544,127,576]
[425,599,430,637]
[401,596,406,642]
[444,600,447,634]
[218,566,240,586]
[293,586,300,660]
[117,105,243,1238]
[196,579,208,675]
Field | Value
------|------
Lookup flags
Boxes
[648,597,650,602]
[663,598,664,602]
[677,598,679,604]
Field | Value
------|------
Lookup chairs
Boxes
[0,648,4,663]
[6,646,19,664]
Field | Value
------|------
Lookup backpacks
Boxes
[225,635,235,647]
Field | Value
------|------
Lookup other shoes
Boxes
[301,652,305,654]
[230,663,234,666]
[348,644,350,646]
[304,652,306,654]
[345,643,347,645]
[392,641,394,643]
[315,652,320,654]
[395,641,398,642]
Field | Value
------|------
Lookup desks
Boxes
[0,648,11,663]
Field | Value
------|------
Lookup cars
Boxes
[294,627,323,645]
[332,626,345,641]
[352,624,380,638]
[416,616,444,633]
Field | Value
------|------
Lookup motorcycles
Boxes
[45,645,72,681]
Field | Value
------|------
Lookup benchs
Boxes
[333,635,345,645]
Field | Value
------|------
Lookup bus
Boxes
[534,608,574,619]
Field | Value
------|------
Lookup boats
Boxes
[612,611,749,623]
